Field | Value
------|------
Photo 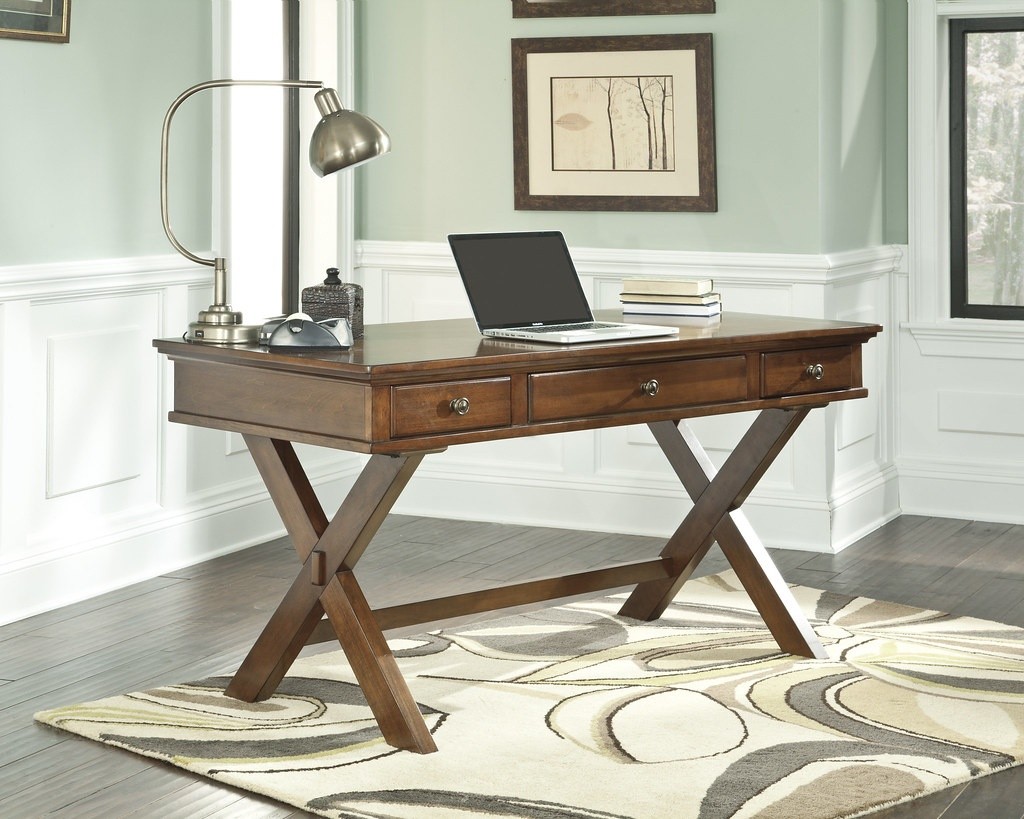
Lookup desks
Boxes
[151,309,883,754]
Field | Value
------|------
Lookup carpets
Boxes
[32,567,1024,819]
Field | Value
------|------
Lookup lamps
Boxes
[162,80,393,344]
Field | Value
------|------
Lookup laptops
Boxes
[447,230,680,342]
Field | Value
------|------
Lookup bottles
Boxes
[302,269,364,335]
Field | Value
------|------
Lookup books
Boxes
[619,279,722,317]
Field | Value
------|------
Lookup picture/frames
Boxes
[0,0,72,43]
[510,32,718,213]
